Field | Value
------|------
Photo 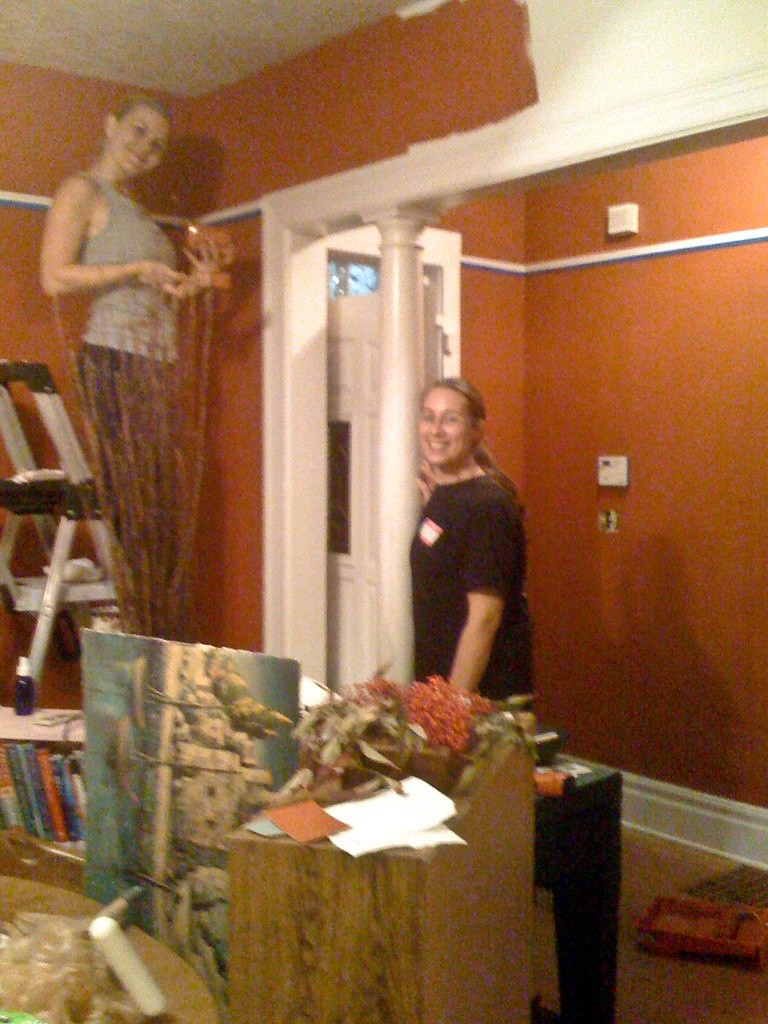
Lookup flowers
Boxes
[300,669,538,788]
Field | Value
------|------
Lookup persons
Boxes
[37,95,236,587]
[408,377,536,708]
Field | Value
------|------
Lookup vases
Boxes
[359,744,461,795]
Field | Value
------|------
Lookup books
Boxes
[0,740,91,850]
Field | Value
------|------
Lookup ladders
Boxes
[0,358,143,718]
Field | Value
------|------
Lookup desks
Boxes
[1,875,216,1023]
[534,752,621,1024]
